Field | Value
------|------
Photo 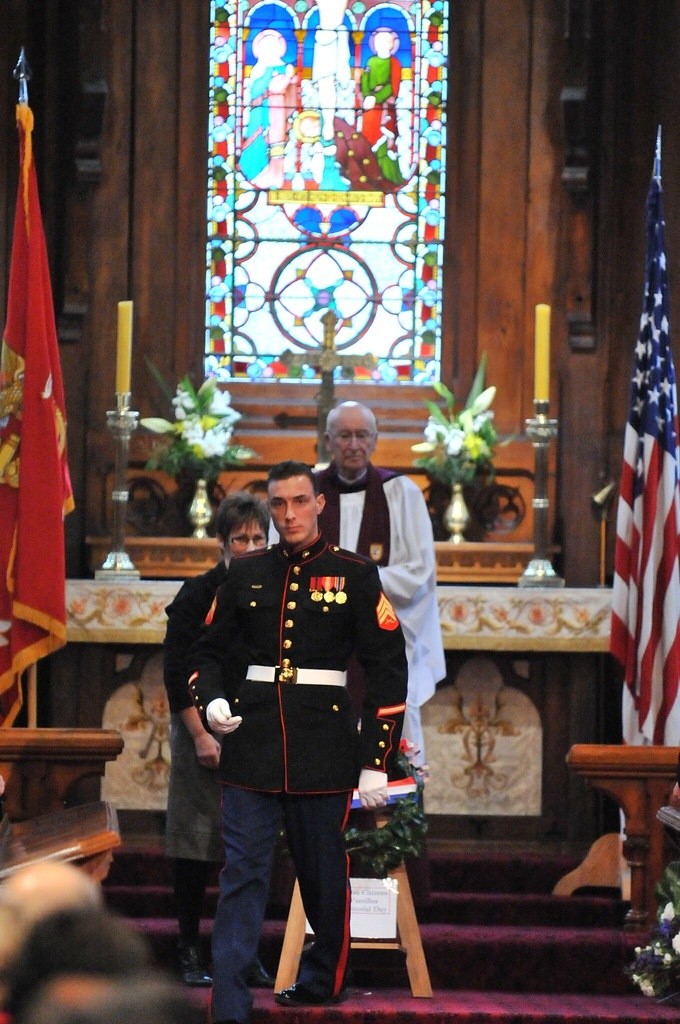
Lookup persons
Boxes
[283,400,446,940]
[0,859,198,1024]
[160,498,280,985]
[199,463,409,1024]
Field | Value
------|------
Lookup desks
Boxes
[85,536,562,582]
[60,579,612,840]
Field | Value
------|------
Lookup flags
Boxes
[0,103,77,803]
[607,123,680,748]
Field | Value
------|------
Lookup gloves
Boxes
[206,697,242,734]
[358,768,389,810]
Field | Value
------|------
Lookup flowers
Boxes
[139,353,262,481]
[625,862,680,1004]
[345,740,429,880]
[410,350,518,487]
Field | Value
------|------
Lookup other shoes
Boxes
[274,982,349,1006]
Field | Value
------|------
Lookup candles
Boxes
[534,302,551,404]
[116,301,132,392]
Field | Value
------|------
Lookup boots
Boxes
[176,947,213,987]
[243,955,276,988]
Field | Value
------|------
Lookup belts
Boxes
[244,665,347,686]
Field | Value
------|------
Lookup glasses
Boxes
[227,535,266,547]
[324,431,375,442]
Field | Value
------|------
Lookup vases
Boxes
[444,483,470,543]
[189,479,213,539]
